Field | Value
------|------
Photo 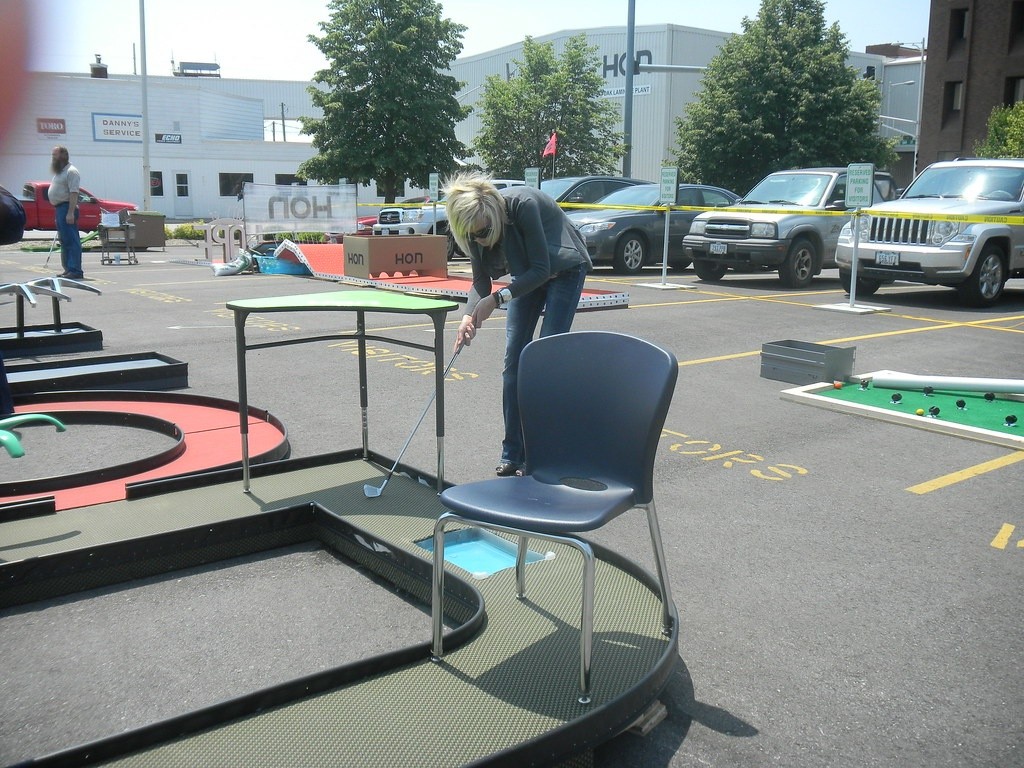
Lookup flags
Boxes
[543,135,556,157]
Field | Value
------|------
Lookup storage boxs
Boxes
[760,337,857,387]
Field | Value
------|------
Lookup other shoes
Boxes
[495,461,520,476]
[516,465,528,476]
[56,271,84,279]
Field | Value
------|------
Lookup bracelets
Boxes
[497,287,512,304]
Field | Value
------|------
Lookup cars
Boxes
[356,196,432,236]
[540,176,656,215]
[562,183,741,277]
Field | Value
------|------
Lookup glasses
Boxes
[461,224,492,243]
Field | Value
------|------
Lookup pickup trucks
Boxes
[16,182,140,233]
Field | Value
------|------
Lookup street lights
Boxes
[886,79,916,140]
[891,36,925,184]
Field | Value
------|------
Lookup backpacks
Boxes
[0,186,26,245]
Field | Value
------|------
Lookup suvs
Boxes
[681,166,895,289]
[834,157,1024,308]
[373,179,527,263]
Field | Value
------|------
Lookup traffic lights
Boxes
[863,65,876,81]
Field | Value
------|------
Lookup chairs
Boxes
[430,329,679,703]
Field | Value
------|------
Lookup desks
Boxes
[225,290,459,496]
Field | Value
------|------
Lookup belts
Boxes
[54,201,69,208]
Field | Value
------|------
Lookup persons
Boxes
[48,147,83,279]
[444,171,594,477]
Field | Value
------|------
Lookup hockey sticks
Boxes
[42,231,57,268]
[363,343,464,498]
